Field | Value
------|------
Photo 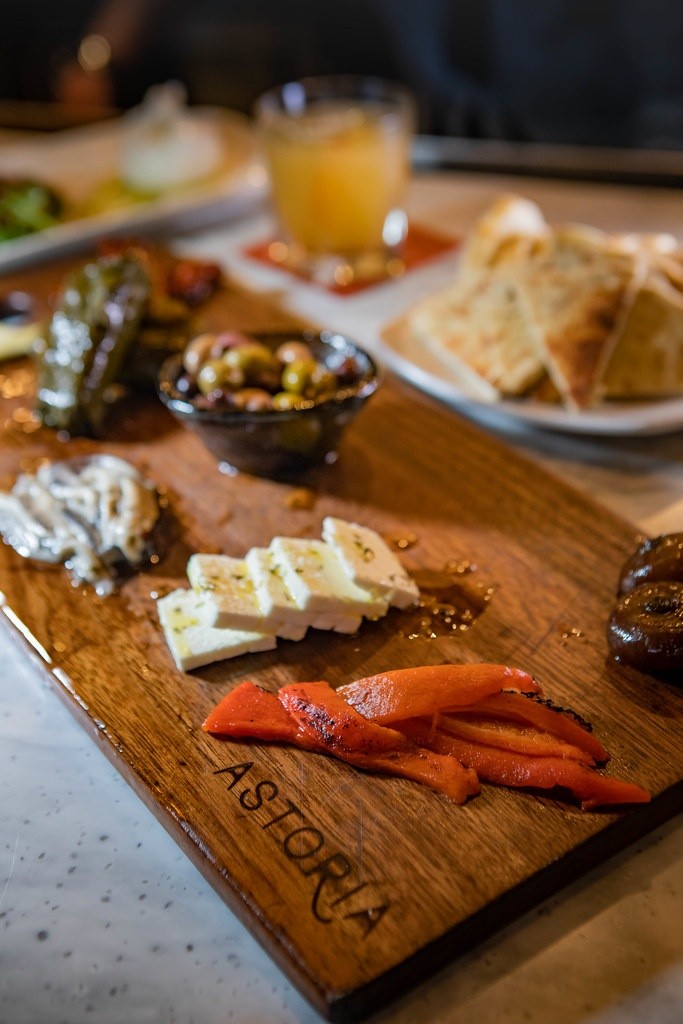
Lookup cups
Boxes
[255,76,417,282]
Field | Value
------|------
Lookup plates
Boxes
[0,109,270,271]
[373,262,683,438]
[1,248,683,1024]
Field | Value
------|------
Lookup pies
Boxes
[413,222,682,412]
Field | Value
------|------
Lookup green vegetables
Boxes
[0,187,61,240]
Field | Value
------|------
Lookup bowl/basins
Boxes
[161,330,381,470]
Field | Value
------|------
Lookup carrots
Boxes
[202,664,650,806]
